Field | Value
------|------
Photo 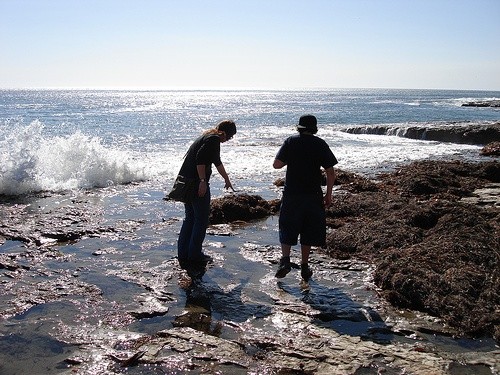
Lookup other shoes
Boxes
[179,254,200,262]
[279,255,291,270]
[300,264,313,277]
[190,255,212,263]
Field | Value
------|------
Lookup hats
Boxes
[298,114,318,128]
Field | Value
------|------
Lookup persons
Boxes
[271,113,339,283]
[169,120,239,276]
